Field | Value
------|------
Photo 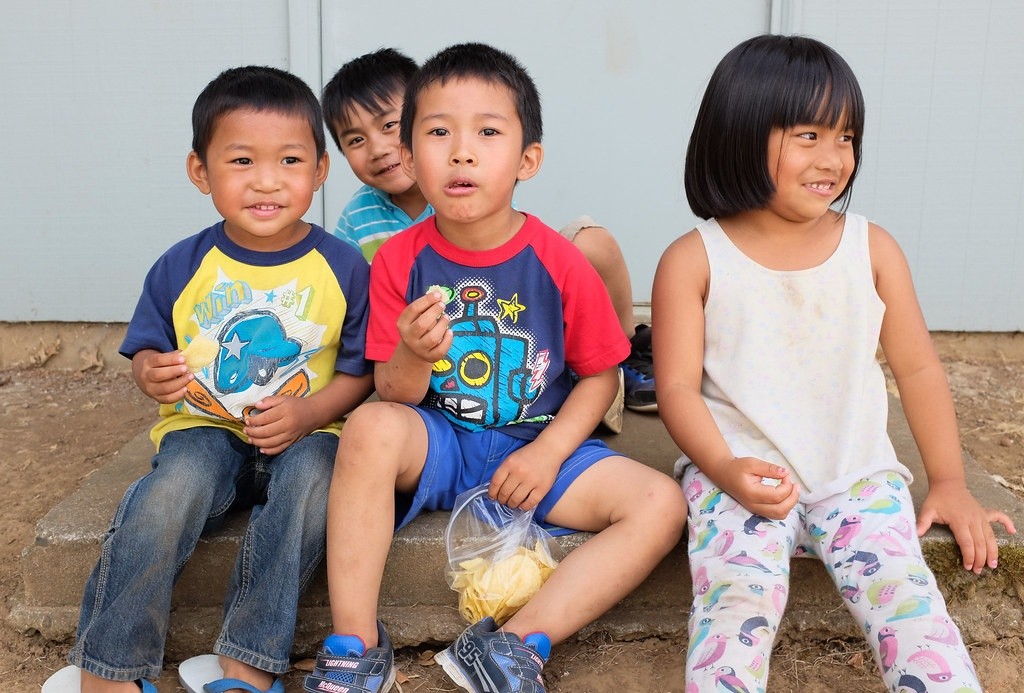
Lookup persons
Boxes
[649,31,1023,693]
[295,47,677,415]
[75,63,378,693]
[302,41,690,693]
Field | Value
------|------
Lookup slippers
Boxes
[41,661,160,693]
[178,652,286,693]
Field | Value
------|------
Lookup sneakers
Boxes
[601,368,625,434]
[616,347,659,411]
[302,618,397,693]
[433,614,550,692]
